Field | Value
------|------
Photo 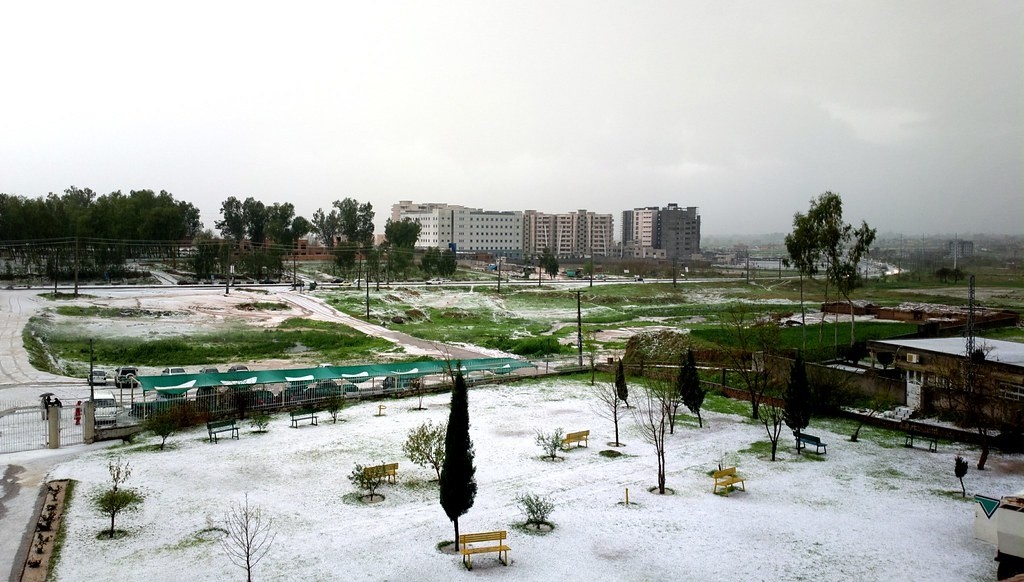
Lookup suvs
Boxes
[114,366,138,388]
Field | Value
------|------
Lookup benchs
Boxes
[460,531,510,570]
[359,463,399,485]
[290,408,319,429]
[561,430,590,452]
[207,418,241,443]
[713,468,745,496]
[904,427,939,452]
[792,430,828,456]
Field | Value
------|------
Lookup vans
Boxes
[86,390,119,426]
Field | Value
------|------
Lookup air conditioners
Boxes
[906,353,919,363]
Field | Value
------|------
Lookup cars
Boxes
[227,364,249,372]
[160,367,187,376]
[381,376,420,389]
[199,367,219,373]
[127,379,360,420]
[87,369,108,386]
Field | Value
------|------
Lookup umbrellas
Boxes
[39,393,54,397]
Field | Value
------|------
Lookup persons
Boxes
[75,401,81,425]
[52,398,61,407]
[39,396,51,420]
